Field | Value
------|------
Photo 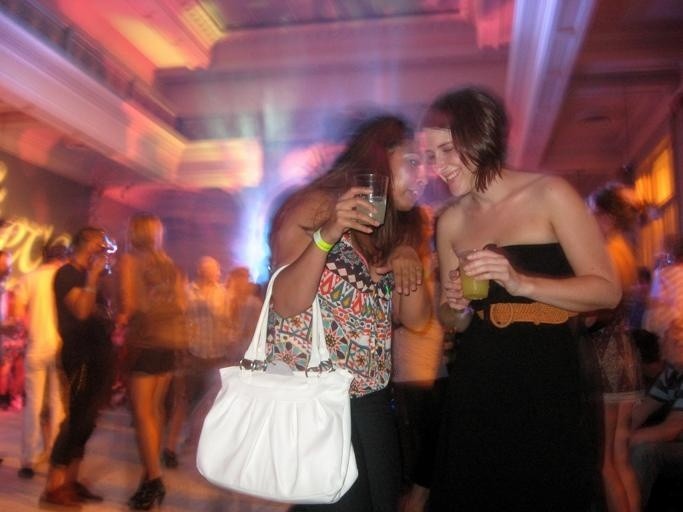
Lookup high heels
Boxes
[127,477,167,511]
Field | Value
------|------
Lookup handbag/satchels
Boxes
[195,263,361,505]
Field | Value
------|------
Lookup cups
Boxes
[460,251,492,300]
[353,173,390,225]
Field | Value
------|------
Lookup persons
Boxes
[256,116,441,510]
[0,184,682,512]
[417,84,627,510]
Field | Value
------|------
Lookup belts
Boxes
[468,302,580,328]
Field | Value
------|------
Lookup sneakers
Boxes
[18,468,104,508]
